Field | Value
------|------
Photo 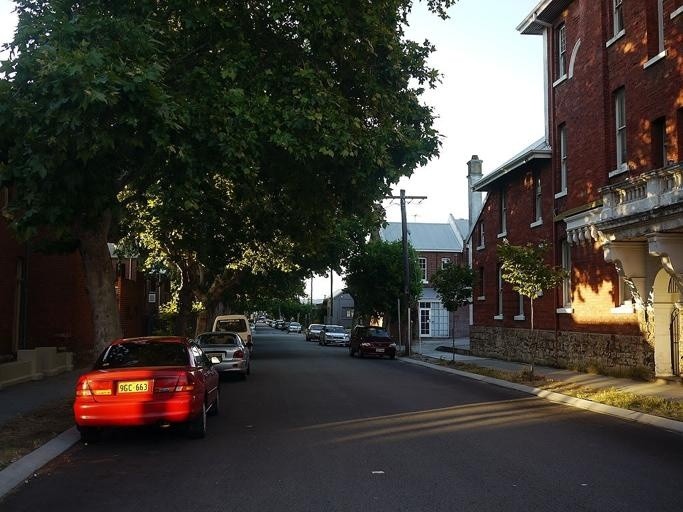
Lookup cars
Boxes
[73,336,219,439]
[265,319,284,330]
[305,324,351,346]
[196,332,250,380]
[287,322,302,333]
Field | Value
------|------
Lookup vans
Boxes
[212,315,254,355]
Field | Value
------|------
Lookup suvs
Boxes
[348,325,396,360]
